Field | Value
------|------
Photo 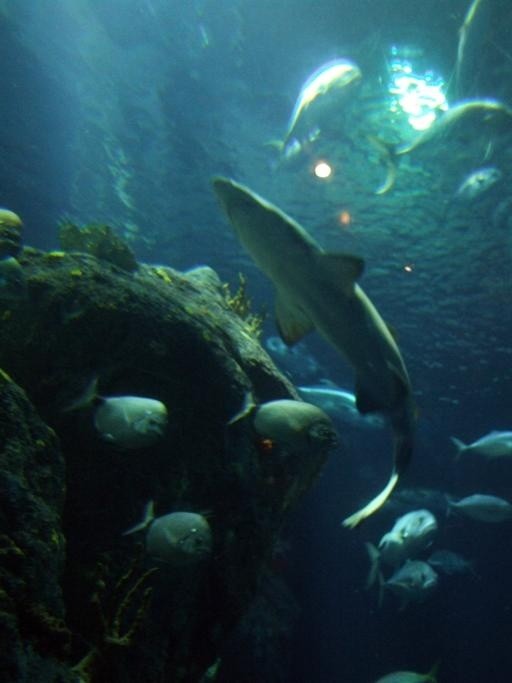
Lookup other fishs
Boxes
[364,95,509,246]
[445,1,494,98]
[264,58,362,187]
